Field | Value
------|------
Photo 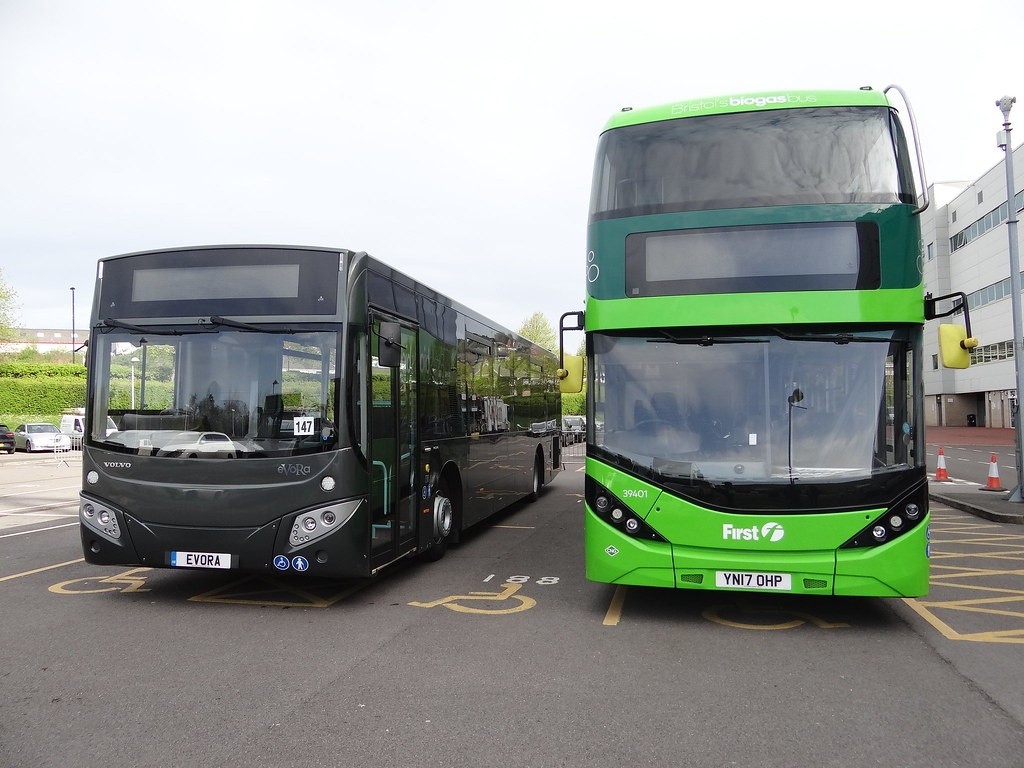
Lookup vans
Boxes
[60,414,118,448]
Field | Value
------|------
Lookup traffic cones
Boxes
[978,453,1009,492]
[930,447,952,481]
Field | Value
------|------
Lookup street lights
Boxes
[71,287,75,364]
[130,357,139,410]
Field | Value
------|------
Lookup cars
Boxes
[562,419,575,447]
[564,417,587,441]
[528,420,559,436]
[11,423,71,453]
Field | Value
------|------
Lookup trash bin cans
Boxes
[967,414,976,427]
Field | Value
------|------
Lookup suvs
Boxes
[156,431,238,460]
[0,424,16,454]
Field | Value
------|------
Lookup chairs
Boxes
[633,392,681,434]
[191,400,249,441]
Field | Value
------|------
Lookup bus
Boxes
[77,245,564,581]
[554,85,979,599]
